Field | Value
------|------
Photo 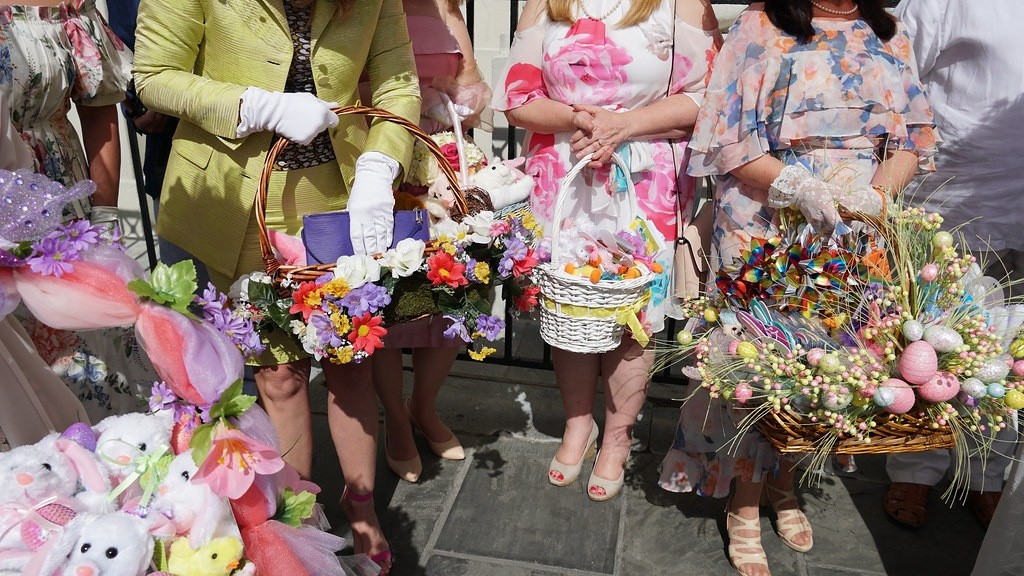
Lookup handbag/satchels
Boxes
[532,152,655,354]
[742,211,960,455]
[437,89,542,287]
[674,201,714,300]
[300,206,430,269]
[255,105,494,353]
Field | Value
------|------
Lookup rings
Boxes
[598,140,603,147]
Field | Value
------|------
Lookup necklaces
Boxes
[580,0,621,21]
[810,0,858,15]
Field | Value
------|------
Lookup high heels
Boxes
[384,416,423,483]
[588,430,633,501]
[406,398,466,460]
[724,492,768,576]
[764,471,813,553]
[548,419,600,486]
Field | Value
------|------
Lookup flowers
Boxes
[1,206,555,499]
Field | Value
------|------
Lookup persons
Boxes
[688,0,1024,576]
[107,0,492,576]
[0,0,164,453]
[488,0,726,501]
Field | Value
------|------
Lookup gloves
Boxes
[347,152,403,258]
[421,101,475,126]
[236,87,340,146]
[768,161,894,240]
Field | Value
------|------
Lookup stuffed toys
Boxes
[535,217,663,284]
[428,156,536,219]
[0,408,256,576]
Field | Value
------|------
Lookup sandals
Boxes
[340,490,392,576]
[883,482,930,527]
[971,490,1001,528]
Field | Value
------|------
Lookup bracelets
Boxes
[870,184,889,196]
